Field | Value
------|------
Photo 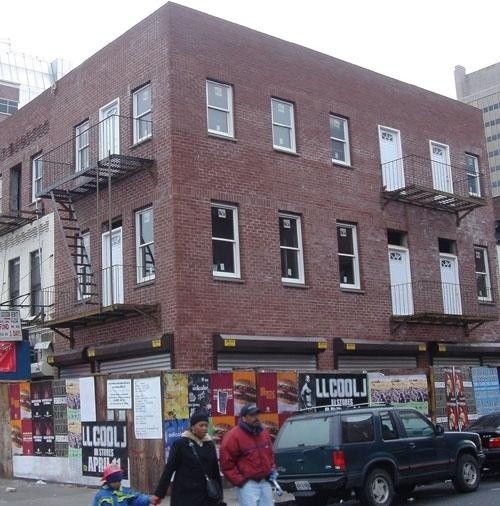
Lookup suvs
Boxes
[271,405,485,505]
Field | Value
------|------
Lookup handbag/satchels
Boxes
[206,479,221,500]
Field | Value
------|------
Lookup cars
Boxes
[464,411,500,478]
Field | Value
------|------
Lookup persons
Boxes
[300,375,313,409]
[152,406,229,506]
[446,374,466,432]
[220,404,280,506]
[373,386,428,403]
[93,463,160,506]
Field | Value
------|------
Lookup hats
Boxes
[190,407,209,425]
[101,464,123,485]
[239,404,261,416]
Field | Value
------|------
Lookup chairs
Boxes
[381,426,397,439]
